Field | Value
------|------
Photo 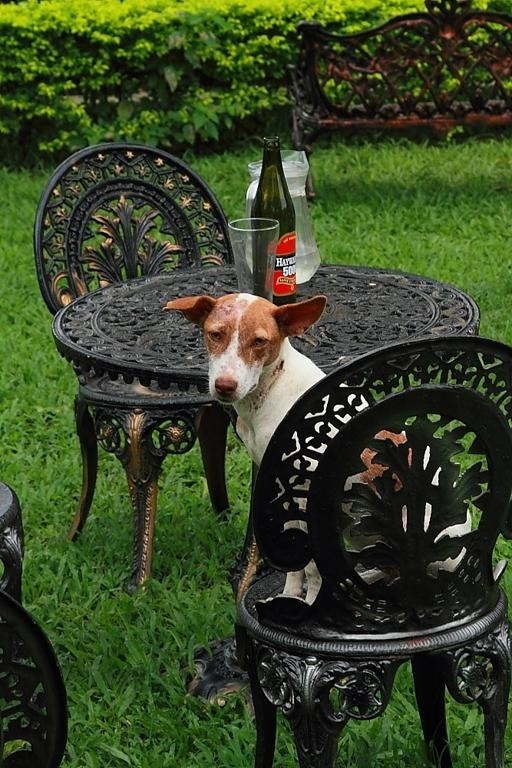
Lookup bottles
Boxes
[251,136,297,304]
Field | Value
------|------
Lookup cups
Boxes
[228,217,280,302]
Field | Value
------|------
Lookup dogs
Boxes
[165,293,472,605]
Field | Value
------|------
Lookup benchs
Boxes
[283,0,512,201]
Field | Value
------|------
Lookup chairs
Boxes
[1,591,69,768]
[33,142,246,596]
[234,335,511,768]
